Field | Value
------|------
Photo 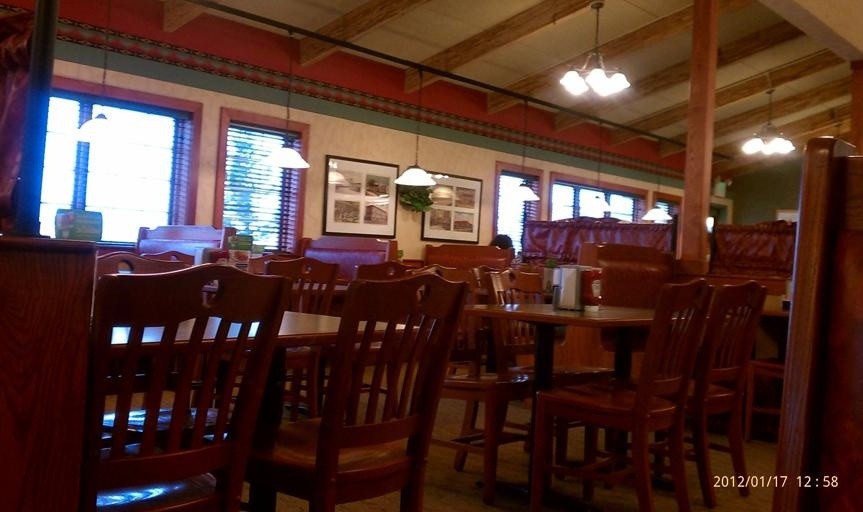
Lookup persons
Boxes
[489,234,511,250]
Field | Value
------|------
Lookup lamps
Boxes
[0,0,675,222]
[559,0,631,97]
[741,89,795,159]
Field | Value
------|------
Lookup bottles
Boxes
[580,268,602,313]
[208,250,229,286]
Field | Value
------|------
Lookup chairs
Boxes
[0,212,797,510]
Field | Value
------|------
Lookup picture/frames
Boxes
[321,154,400,239]
[421,169,485,244]
